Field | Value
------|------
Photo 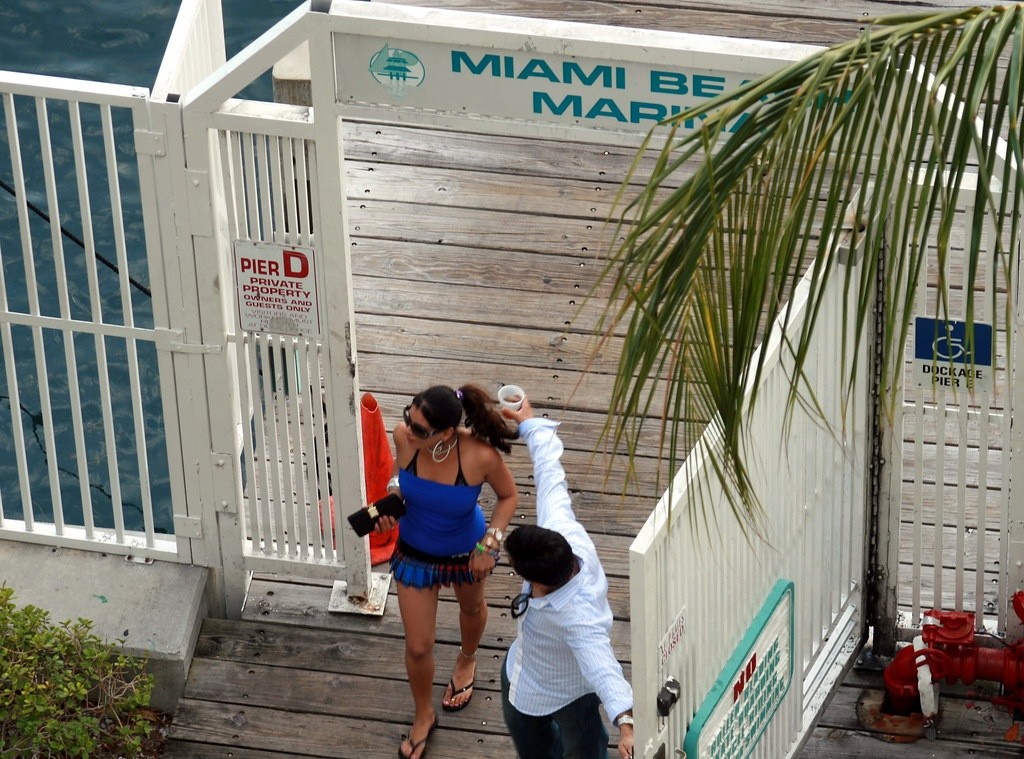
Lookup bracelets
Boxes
[481,533,501,553]
[475,542,499,561]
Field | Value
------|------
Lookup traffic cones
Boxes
[361,393,399,567]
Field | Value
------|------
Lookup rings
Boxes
[391,525,395,529]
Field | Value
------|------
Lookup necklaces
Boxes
[426,432,459,456]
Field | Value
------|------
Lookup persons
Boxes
[501,392,636,759]
[373,383,520,759]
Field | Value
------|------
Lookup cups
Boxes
[498,385,525,421]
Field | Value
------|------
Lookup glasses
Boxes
[403,406,440,440]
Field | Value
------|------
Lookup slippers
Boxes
[442,666,477,711]
[400,711,437,759]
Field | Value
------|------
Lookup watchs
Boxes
[485,527,504,543]
[617,714,633,727]
